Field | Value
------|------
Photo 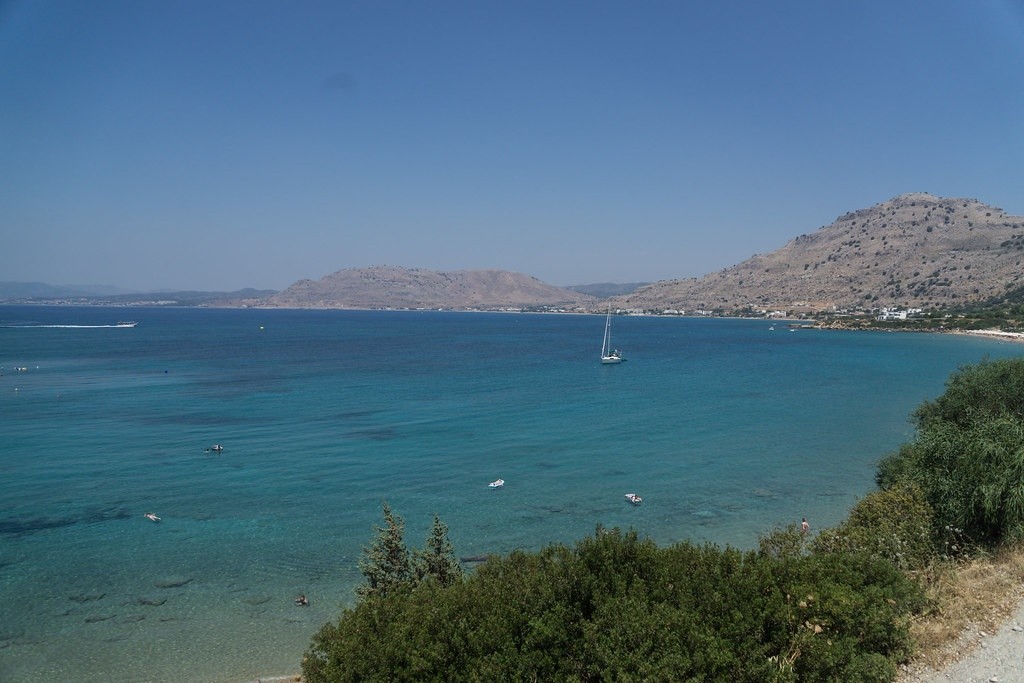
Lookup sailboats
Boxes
[600,301,623,363]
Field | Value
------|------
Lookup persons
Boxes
[144,513,161,521]
[631,495,642,503]
[205,445,222,455]
[491,479,503,486]
[295,596,308,606]
[801,518,809,531]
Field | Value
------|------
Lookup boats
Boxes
[117,321,139,327]
[626,493,642,504]
[488,479,505,489]
